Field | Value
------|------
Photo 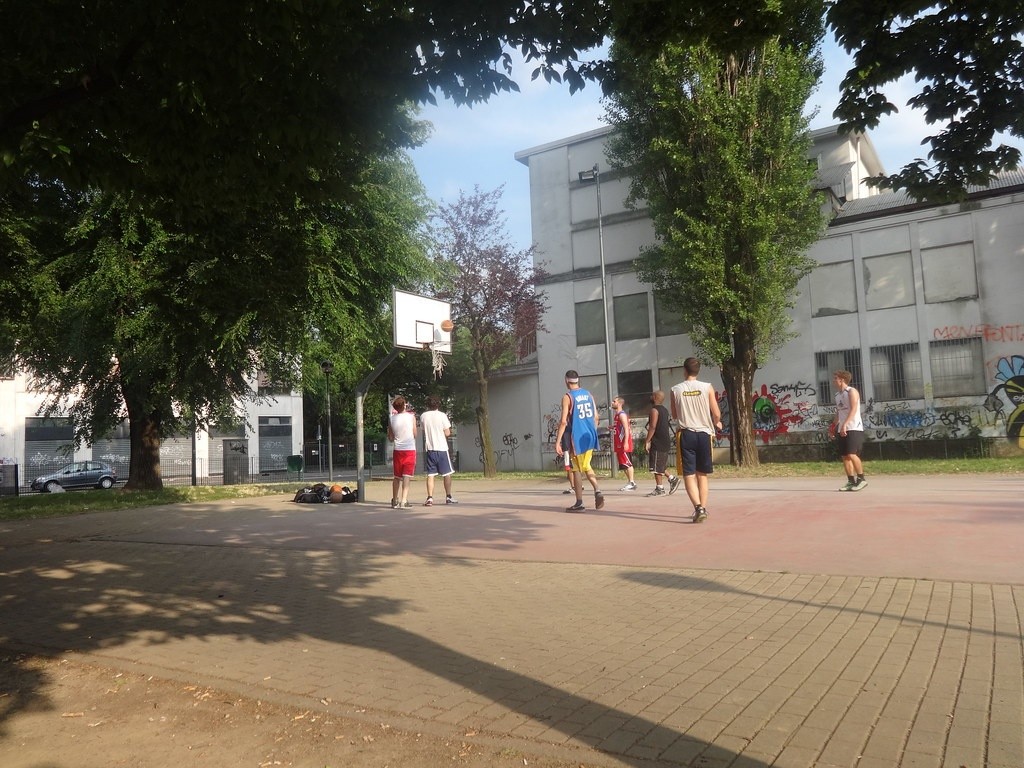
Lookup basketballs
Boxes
[331,485,342,493]
[330,491,343,503]
[441,321,454,332]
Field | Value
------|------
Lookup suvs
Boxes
[32,460,116,492]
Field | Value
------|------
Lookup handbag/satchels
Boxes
[294,484,358,503]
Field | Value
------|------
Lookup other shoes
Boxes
[566,504,585,512]
[692,505,708,522]
[851,477,868,491]
[647,486,666,497]
[595,491,604,509]
[620,481,637,491]
[446,496,458,504]
[668,476,681,494]
[839,479,855,491]
[425,497,433,505]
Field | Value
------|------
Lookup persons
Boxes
[387,397,418,509]
[606,397,638,492]
[420,394,458,507]
[645,390,681,498]
[828,369,869,492]
[669,356,722,524]
[555,370,605,513]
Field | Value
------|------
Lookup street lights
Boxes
[320,361,335,484]
